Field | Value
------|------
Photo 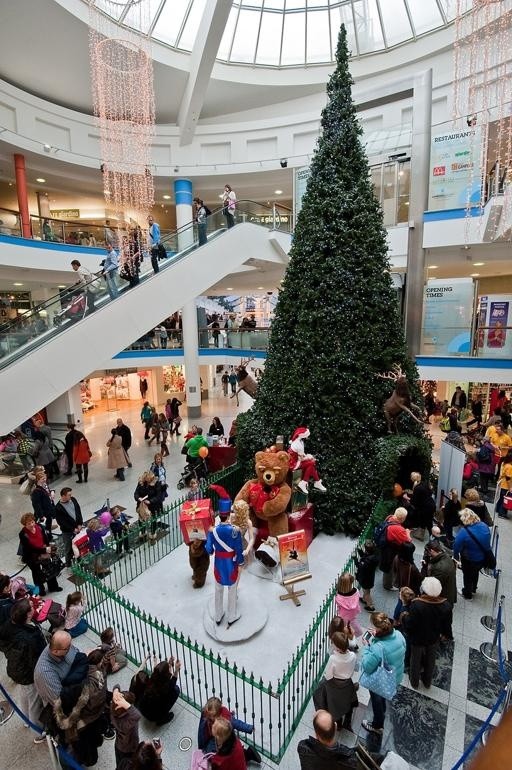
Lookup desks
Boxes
[202,389,208,399]
[164,392,185,402]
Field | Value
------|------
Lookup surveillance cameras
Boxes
[267,291,272,295]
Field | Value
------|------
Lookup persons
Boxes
[200,377,204,402]
[1,574,255,767]
[163,371,185,392]
[71,259,97,316]
[2,411,92,483]
[297,442,495,767]
[218,184,236,230]
[222,371,237,396]
[184,417,224,470]
[1,454,169,594]
[288,428,327,495]
[106,418,132,481]
[141,398,182,458]
[103,197,210,299]
[205,498,245,628]
[42,219,52,242]
[140,374,148,399]
[423,382,511,520]
[132,311,257,349]
[487,321,505,348]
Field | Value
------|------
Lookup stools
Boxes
[287,468,306,512]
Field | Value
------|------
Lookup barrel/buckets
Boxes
[501,491,512,510]
[212,434,219,446]
[217,498,233,513]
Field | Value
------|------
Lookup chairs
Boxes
[255,503,313,549]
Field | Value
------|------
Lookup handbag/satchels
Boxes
[137,501,152,523]
[6,647,37,685]
[358,642,397,702]
[418,495,436,512]
[484,501,493,527]
[72,528,90,559]
[476,446,491,465]
[18,477,33,494]
[38,553,65,581]
[483,547,496,570]
[191,749,217,770]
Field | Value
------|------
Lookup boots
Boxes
[145,431,181,457]
[148,532,157,546]
[137,530,147,544]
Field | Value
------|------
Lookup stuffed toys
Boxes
[189,540,211,588]
[230,451,291,567]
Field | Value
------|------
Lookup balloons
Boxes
[391,483,404,497]
[199,446,208,459]
[100,512,112,524]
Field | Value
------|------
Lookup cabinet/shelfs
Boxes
[469,386,512,406]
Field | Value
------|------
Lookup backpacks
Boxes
[370,514,400,547]
[439,413,452,432]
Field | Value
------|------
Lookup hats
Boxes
[420,576,442,598]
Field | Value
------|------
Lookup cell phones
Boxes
[363,631,372,641]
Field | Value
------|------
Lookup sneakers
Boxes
[383,584,399,591]
[38,587,63,596]
[359,597,375,611]
[63,470,88,483]
[246,745,261,764]
[155,711,174,727]
[457,586,476,602]
[361,719,384,735]
[33,734,49,745]
[119,549,133,559]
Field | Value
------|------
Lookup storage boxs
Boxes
[179,497,213,544]
